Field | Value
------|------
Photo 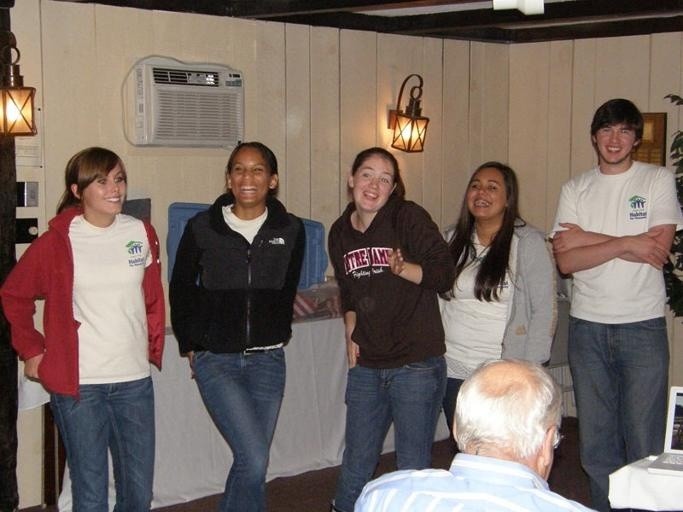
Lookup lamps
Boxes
[0,32,38,135]
[387,73,430,155]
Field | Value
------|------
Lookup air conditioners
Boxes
[130,63,245,149]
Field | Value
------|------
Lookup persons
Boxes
[355,357,590,511]
[546,97,683,511]
[327,147,457,512]
[167,141,306,512]
[0,147,165,512]
[437,160,559,457]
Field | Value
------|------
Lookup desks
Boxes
[610,455,683,512]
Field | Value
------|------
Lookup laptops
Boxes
[646,385,683,478]
